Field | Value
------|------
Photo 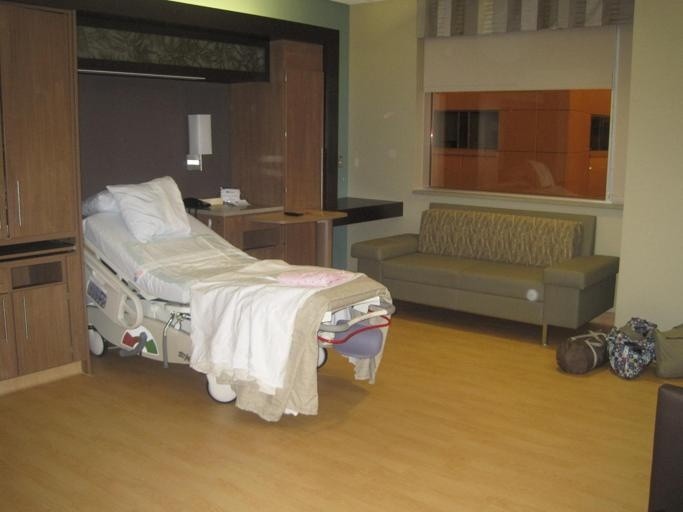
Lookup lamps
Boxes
[183,110,213,173]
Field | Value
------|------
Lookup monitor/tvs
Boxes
[648,383,683,512]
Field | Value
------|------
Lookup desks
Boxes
[250,208,347,267]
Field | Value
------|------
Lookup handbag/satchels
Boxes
[607,318,655,380]
[656,321,682,379]
[556,331,607,376]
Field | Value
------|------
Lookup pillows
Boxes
[107,176,190,243]
[78,184,120,218]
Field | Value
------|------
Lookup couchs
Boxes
[346,196,619,348]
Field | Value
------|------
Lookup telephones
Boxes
[183,197,211,209]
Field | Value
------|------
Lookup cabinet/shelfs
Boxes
[0,241,94,400]
[225,39,327,210]
[1,0,84,252]
[186,202,282,263]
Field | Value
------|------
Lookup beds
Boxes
[77,178,399,425]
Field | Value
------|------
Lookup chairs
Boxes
[645,377,681,511]
